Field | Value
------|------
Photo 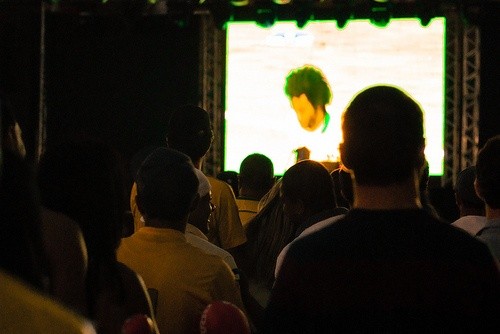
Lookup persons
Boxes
[262,86,499,334]
[284,66,332,135]
[0,100,500,334]
